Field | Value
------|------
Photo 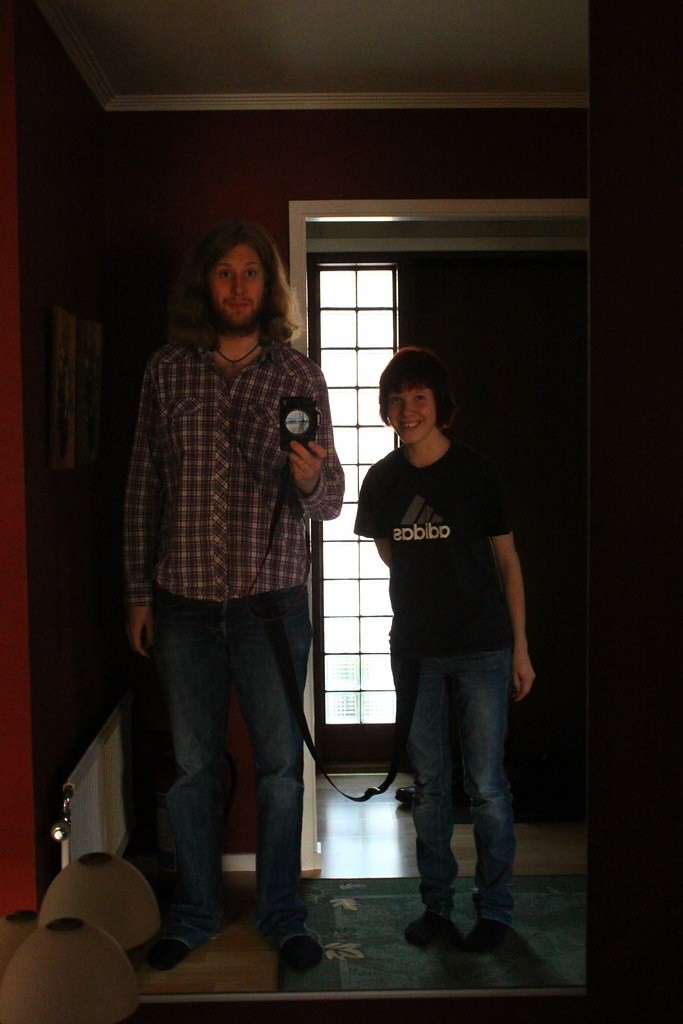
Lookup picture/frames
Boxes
[44,303,103,472]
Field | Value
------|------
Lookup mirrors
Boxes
[11,0,598,1001]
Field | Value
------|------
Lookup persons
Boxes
[354,345,536,954]
[123,217,344,978]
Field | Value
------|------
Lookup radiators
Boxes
[49,691,128,876]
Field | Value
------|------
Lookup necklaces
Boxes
[216,345,259,362]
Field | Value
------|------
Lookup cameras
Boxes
[280,396,322,452]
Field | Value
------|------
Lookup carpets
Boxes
[278,873,586,993]
